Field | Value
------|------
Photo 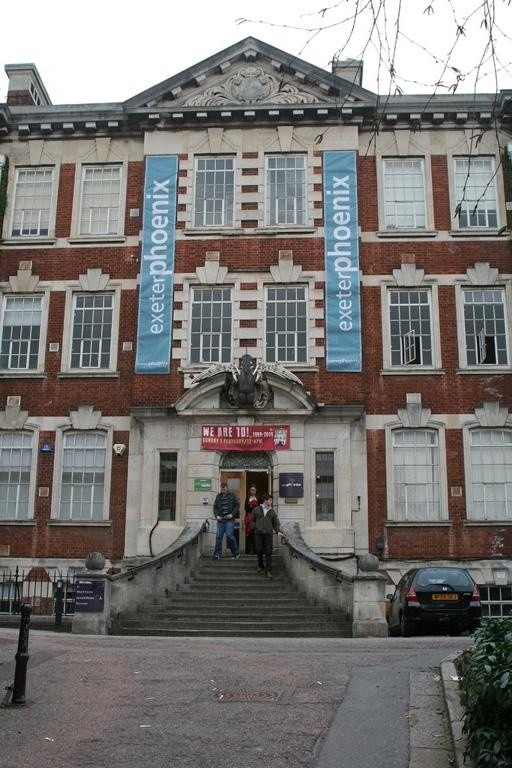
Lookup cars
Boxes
[386,565,483,638]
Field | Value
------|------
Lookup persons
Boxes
[250,494,284,579]
[243,483,263,555]
[213,482,241,561]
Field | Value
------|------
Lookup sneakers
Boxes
[215,554,219,559]
[267,571,272,578]
[257,565,264,572]
[232,554,240,560]
